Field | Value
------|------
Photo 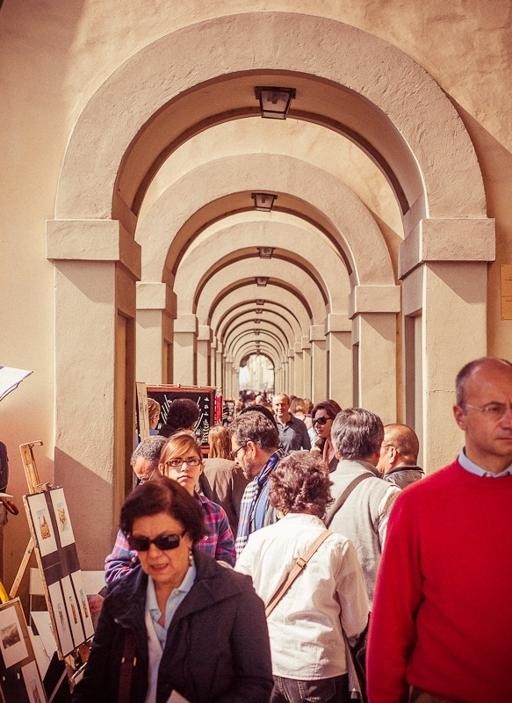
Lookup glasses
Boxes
[230,439,259,457]
[463,398,509,422]
[311,414,334,426]
[164,456,202,467]
[125,530,181,552]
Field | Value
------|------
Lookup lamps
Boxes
[0,365,36,404]
[251,192,278,213]
[254,276,270,286]
[255,247,276,258]
[253,85,295,119]
[255,299,264,356]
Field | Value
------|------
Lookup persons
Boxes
[365,354,512,700]
[82,389,421,703]
